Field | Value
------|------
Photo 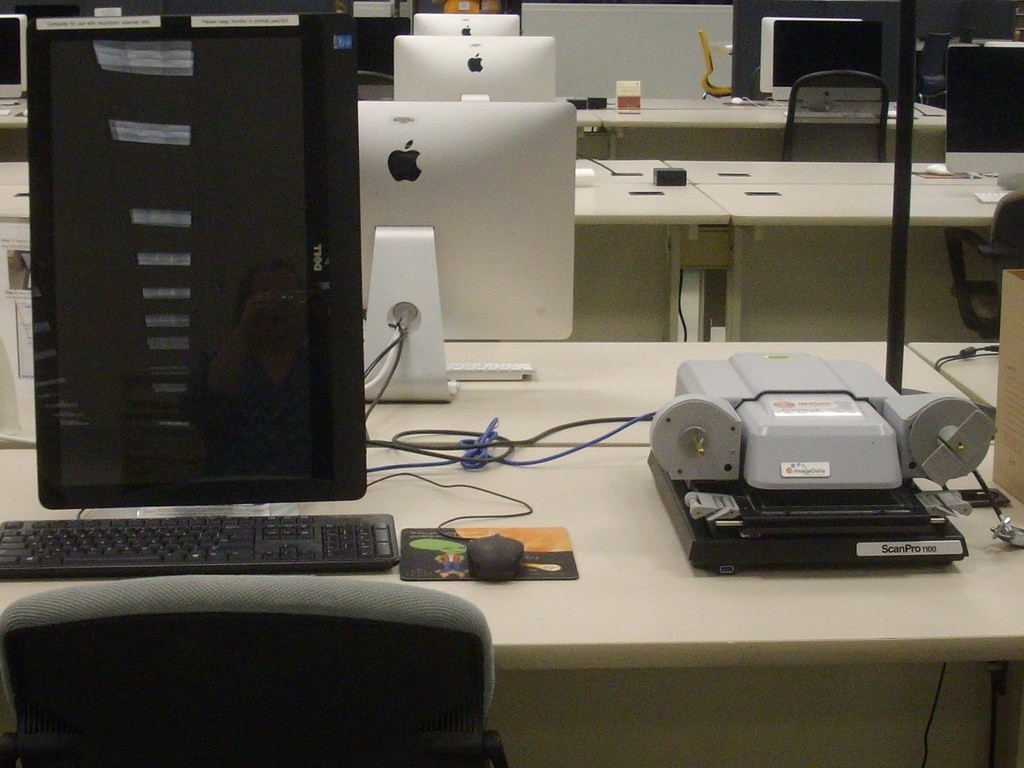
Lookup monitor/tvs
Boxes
[945,46,1024,190]
[760,17,883,107]
[0,0,577,510]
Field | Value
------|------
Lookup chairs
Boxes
[959,24,976,43]
[781,71,888,163]
[915,31,953,111]
[0,575,512,768]
[697,29,731,99]
[944,189,1024,342]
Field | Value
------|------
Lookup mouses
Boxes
[888,110,897,118]
[732,97,745,104]
[468,533,523,581]
[926,164,951,176]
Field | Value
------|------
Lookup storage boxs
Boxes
[992,269,1024,505]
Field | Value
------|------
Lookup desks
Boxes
[0,94,1024,768]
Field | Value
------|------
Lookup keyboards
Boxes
[974,191,1011,203]
[0,515,400,580]
[784,111,875,119]
[0,109,11,115]
[445,362,535,380]
